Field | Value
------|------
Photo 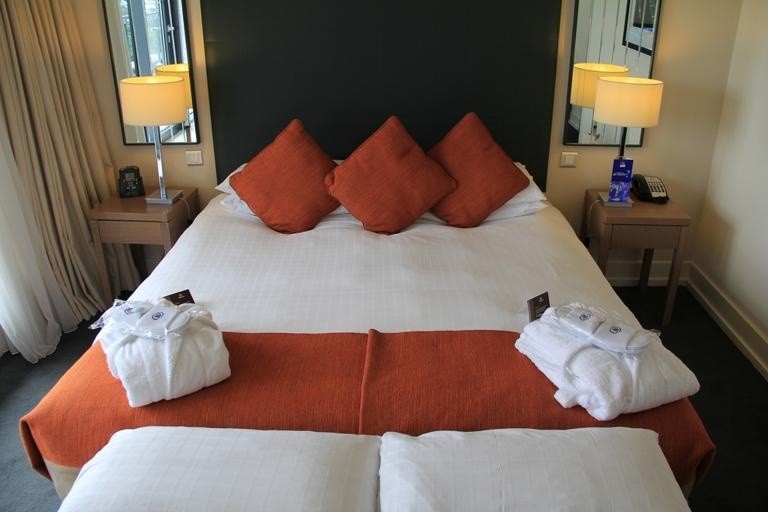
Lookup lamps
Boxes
[569,63,630,143]
[594,77,663,208]
[154,63,195,144]
[119,75,189,203]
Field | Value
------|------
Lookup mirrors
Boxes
[102,0,203,145]
[563,2,663,148]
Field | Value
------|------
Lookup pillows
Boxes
[324,115,457,236]
[378,429,696,512]
[424,114,530,229]
[54,428,381,510]
[230,115,342,237]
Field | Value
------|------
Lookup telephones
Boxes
[632,175,667,203]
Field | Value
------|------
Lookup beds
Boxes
[25,160,717,506]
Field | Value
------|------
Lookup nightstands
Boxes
[87,185,198,309]
[583,188,692,334]
